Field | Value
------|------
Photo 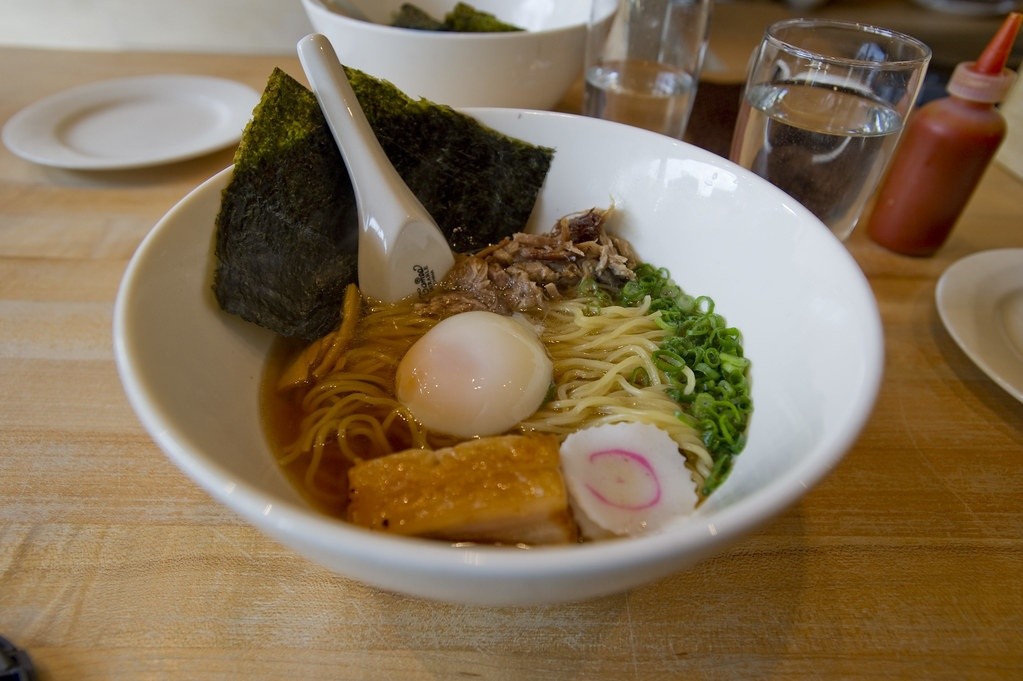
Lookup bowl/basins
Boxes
[113,109,889,610]
[303,0,624,110]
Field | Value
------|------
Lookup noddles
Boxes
[254,198,753,547]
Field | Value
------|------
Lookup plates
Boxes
[2,74,263,172]
[932,247,1023,402]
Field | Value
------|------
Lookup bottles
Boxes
[863,10,1023,260]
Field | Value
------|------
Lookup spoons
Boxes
[296,31,455,306]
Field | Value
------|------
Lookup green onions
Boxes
[572,263,752,498]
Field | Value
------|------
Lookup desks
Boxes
[0,45,1022,681]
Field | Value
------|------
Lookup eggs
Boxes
[392,310,555,440]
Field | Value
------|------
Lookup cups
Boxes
[581,0,714,141]
[725,19,935,244]
